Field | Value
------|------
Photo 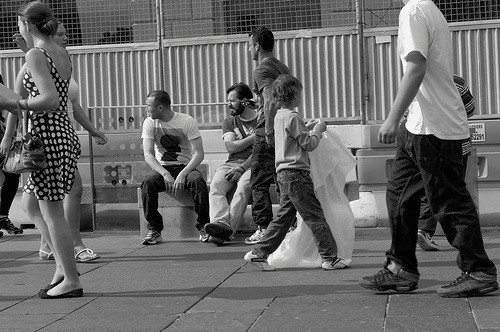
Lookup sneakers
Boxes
[199,228,210,242]
[360,267,418,294]
[437,272,500,298]
[143,230,162,244]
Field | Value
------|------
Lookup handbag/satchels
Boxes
[2,99,47,174]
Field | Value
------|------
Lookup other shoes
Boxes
[244,229,266,244]
[38,289,84,299]
[321,256,352,270]
[417,229,439,251]
[244,250,276,271]
[208,236,224,246]
[0,217,23,235]
[45,275,64,291]
[204,222,233,238]
[0,231,4,237]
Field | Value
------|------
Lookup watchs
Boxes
[264,132,274,139]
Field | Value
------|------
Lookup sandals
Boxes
[39,249,55,261]
[75,247,101,262]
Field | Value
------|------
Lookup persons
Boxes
[0,0,110,299]
[358,0,500,297]
[243,73,354,272]
[141,88,210,246]
[203,81,258,246]
[241,25,297,245]
